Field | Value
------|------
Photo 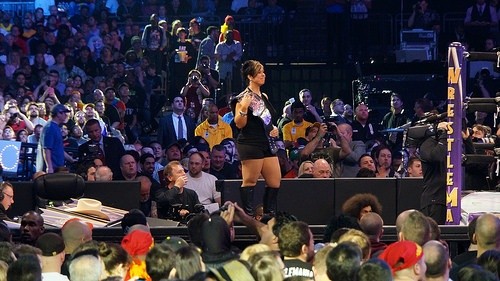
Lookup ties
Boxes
[97,143,105,157]
[178,115,183,138]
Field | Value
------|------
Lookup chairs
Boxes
[34,173,86,213]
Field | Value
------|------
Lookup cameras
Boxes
[192,76,198,82]
[202,63,207,68]
[413,2,422,9]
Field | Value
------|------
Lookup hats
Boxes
[305,121,321,135]
[163,236,189,249]
[378,241,423,274]
[122,209,145,232]
[65,198,110,223]
[164,142,181,154]
[202,217,234,262]
[35,234,65,257]
[50,104,72,117]
[83,103,95,110]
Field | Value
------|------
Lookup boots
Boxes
[264,186,280,216]
[241,185,256,218]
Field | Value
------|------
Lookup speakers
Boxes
[7,182,34,219]
[276,178,334,225]
[334,178,396,225]
[85,180,141,211]
[397,177,424,214]
[214,179,267,213]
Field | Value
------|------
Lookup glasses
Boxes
[2,191,14,200]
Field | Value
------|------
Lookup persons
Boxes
[420,123,467,225]
[155,161,204,223]
[183,152,221,207]
[230,61,283,219]
[0,0,500,218]
[0,182,500,281]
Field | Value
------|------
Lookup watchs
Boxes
[208,73,211,76]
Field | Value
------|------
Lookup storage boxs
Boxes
[396,28,438,62]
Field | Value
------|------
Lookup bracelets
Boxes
[198,83,201,85]
[239,111,247,117]
